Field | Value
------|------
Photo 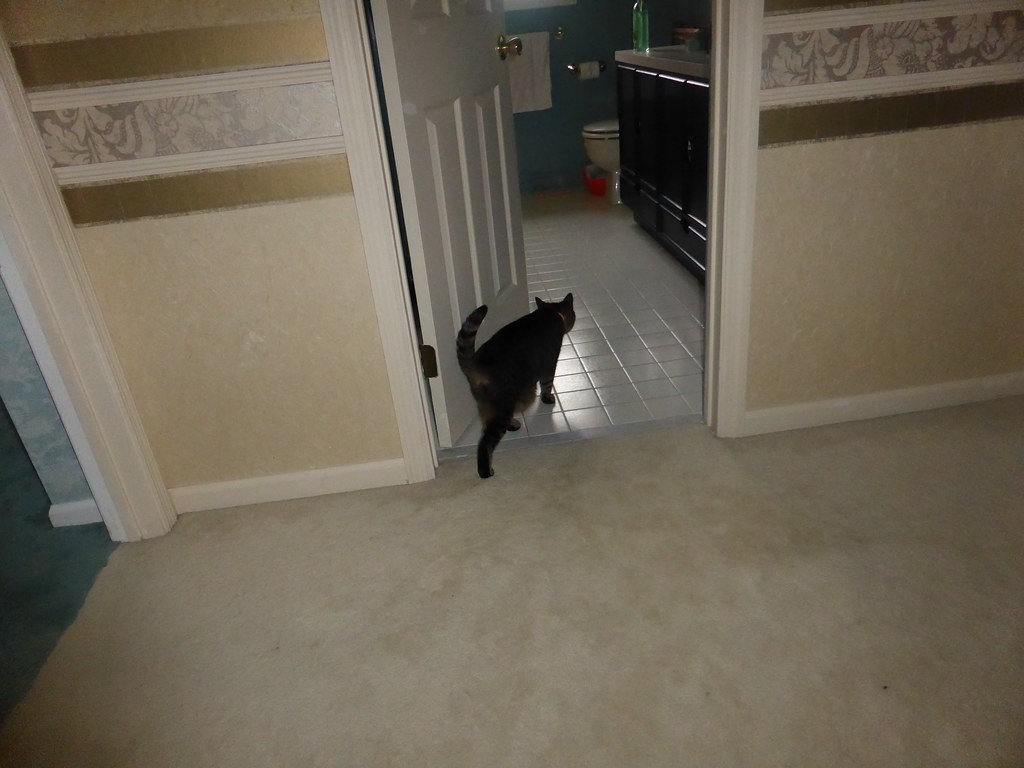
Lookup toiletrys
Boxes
[632,0,649,57]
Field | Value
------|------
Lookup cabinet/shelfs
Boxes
[615,57,711,286]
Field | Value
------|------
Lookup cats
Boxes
[456,291,577,480]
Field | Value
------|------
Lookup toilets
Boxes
[581,116,632,206]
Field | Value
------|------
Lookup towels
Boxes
[504,30,553,113]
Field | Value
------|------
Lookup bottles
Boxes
[633,0,651,52]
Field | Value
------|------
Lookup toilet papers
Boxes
[577,60,601,81]
